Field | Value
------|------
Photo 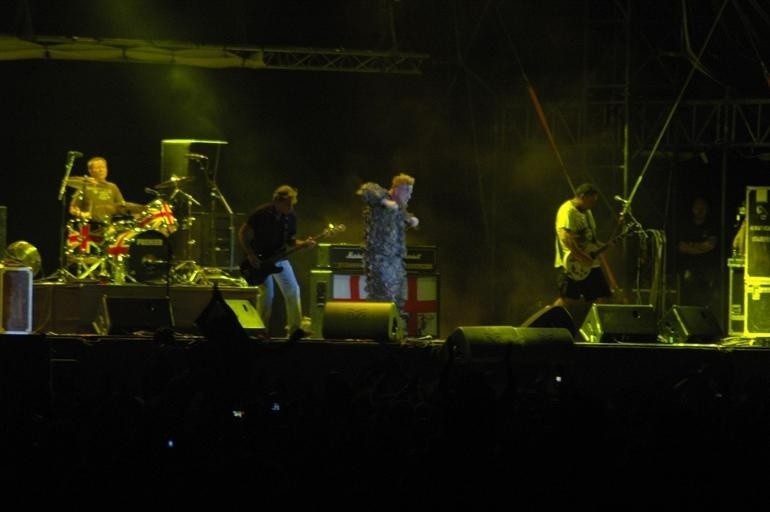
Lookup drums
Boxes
[107,229,169,282]
[135,199,178,237]
[66,218,112,263]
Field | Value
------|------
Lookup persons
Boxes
[554,182,618,343]
[675,193,721,311]
[357,171,422,314]
[67,156,144,230]
[237,184,320,342]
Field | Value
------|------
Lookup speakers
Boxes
[195,297,266,340]
[322,300,404,342]
[578,302,659,344]
[519,303,575,331]
[656,304,724,343]
[92,295,176,334]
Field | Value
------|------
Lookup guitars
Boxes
[241,224,345,285]
[563,223,637,281]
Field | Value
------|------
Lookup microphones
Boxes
[183,153,208,161]
[68,151,84,158]
[614,195,627,205]
[142,258,168,267]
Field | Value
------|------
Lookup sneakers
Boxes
[293,328,312,338]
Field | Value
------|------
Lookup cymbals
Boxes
[155,177,192,189]
[62,177,106,188]
